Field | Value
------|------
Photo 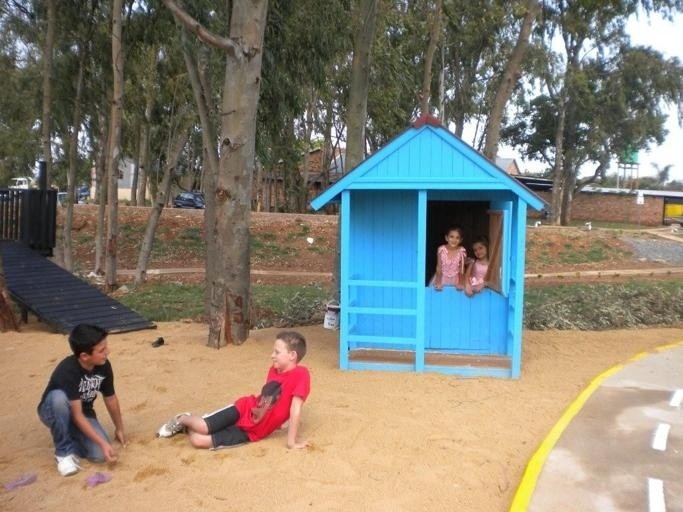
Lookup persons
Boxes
[156,332,310,449]
[429,226,468,289]
[37,324,126,476]
[463,234,489,296]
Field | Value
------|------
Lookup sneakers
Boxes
[157,412,191,438]
[55,454,85,477]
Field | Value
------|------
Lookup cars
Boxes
[170,190,207,209]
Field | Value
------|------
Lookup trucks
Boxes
[8,176,90,207]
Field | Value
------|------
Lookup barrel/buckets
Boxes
[323,298,340,329]
